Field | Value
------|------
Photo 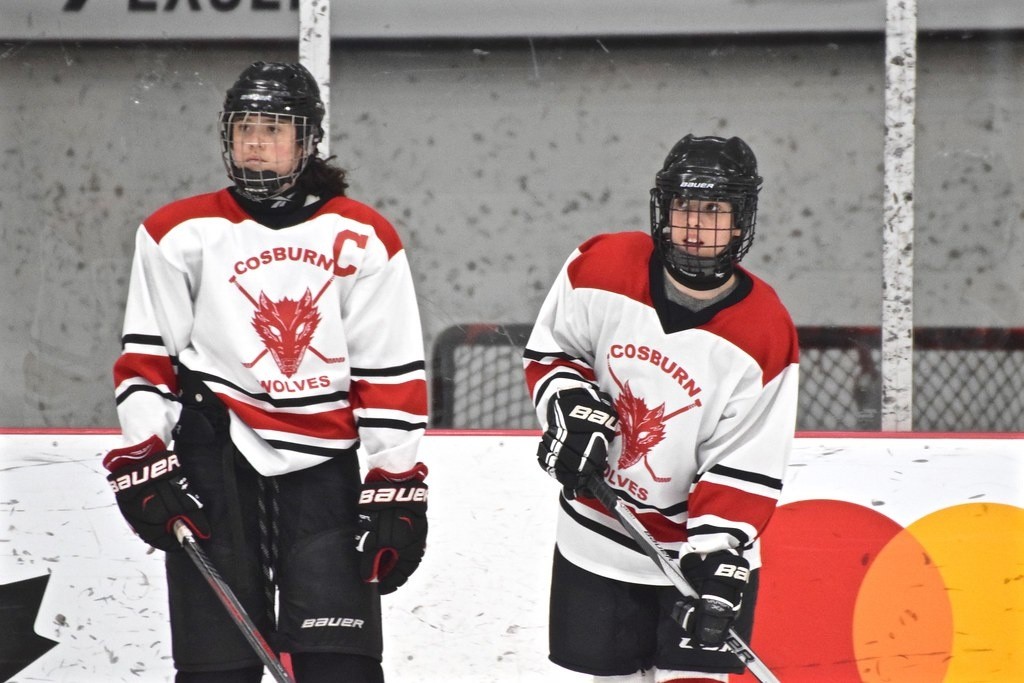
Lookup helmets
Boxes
[218,60,325,204]
[650,132,764,292]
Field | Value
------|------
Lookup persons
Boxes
[101,59,430,683]
[523,133,800,683]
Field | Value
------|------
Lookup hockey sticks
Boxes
[585,472,781,682]
[170,517,292,682]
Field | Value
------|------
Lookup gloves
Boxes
[103,436,212,555]
[533,387,619,500]
[354,461,427,596]
[668,549,751,652]
